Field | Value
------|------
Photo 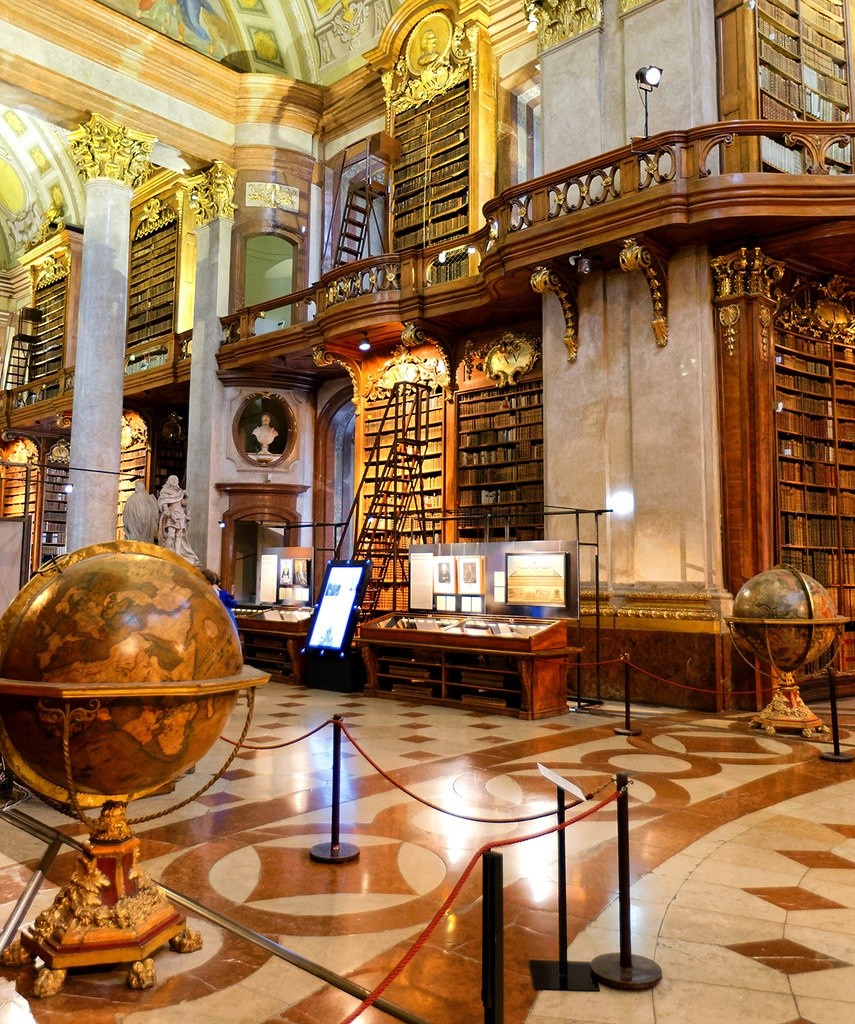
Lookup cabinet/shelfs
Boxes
[0,458,69,575]
[363,385,446,612]
[389,61,471,291]
[237,628,308,686]
[770,322,855,686]
[453,376,545,542]
[126,216,179,366]
[27,278,68,406]
[115,446,147,540]
[353,638,584,720]
[714,0,855,175]
[149,432,187,499]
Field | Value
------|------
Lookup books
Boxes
[116,447,147,541]
[125,221,177,376]
[461,671,507,708]
[254,638,283,676]
[770,327,855,675]
[358,392,442,611]
[392,84,470,289]
[42,467,69,558]
[28,277,67,402]
[388,665,433,697]
[756,0,853,173]
[454,380,545,544]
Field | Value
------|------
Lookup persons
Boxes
[201,568,239,636]
[439,563,450,583]
[157,475,191,554]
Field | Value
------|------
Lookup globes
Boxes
[723,564,851,736]
[2,535,278,1000]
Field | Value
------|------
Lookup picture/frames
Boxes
[293,558,309,586]
[231,390,297,468]
[433,556,456,594]
[456,556,483,596]
[504,551,569,609]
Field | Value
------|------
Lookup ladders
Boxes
[333,382,432,616]
[1,307,43,396]
[321,129,402,268]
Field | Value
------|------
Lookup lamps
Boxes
[569,254,591,275]
[635,65,663,139]
[358,331,370,350]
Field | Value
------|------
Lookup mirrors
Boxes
[214,482,313,604]
